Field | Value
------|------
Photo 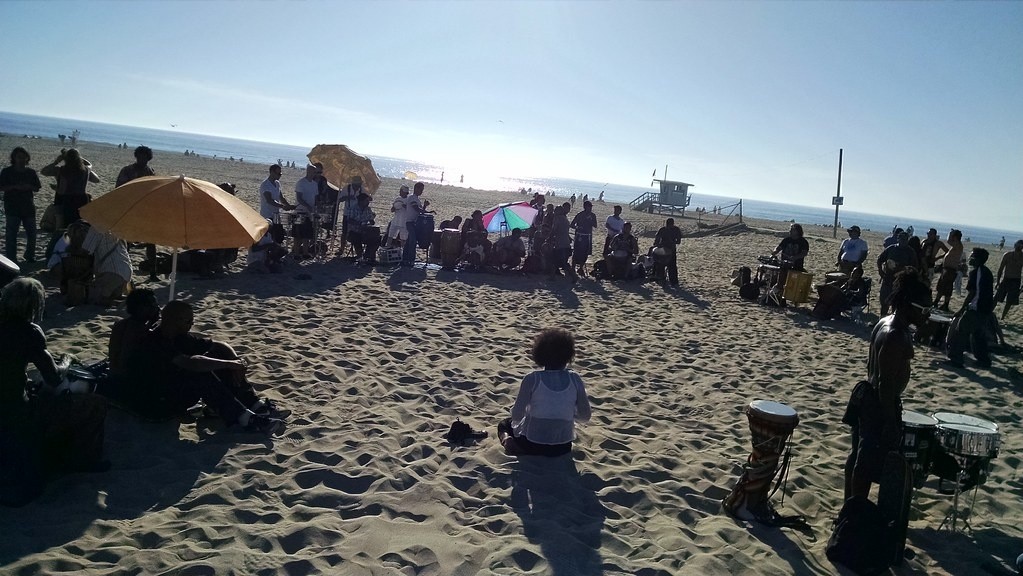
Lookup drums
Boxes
[813,284,847,318]
[825,271,847,281]
[928,411,999,484]
[757,264,781,275]
[935,423,1002,458]
[898,408,936,477]
[574,231,592,254]
[429,229,443,259]
[863,277,872,294]
[417,213,434,249]
[611,251,628,280]
[721,400,800,522]
[440,228,461,272]
[310,212,332,225]
[290,210,311,225]
[782,268,813,303]
[770,260,794,289]
[312,205,333,214]
[272,211,293,225]
[913,314,952,347]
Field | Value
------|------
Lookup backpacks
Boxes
[825,494,896,576]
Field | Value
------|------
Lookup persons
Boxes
[835,223,870,275]
[599,190,604,201]
[499,326,591,459]
[875,224,1023,367]
[260,159,376,260]
[604,205,639,280]
[0,274,106,475]
[844,265,935,503]
[41,135,239,310]
[440,171,444,183]
[382,182,597,283]
[773,222,809,295]
[105,281,290,436]
[654,218,681,284]
[696,204,721,214]
[460,174,464,183]
[0,148,39,266]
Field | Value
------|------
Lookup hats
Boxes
[401,183,409,193]
[350,177,363,185]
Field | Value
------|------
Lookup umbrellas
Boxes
[77,172,269,301]
[306,144,382,244]
[405,170,418,179]
[482,199,537,237]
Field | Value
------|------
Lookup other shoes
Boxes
[446,422,485,443]
[65,457,111,473]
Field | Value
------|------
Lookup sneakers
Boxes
[254,404,290,420]
[243,414,282,434]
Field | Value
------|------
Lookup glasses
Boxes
[317,171,322,174]
[910,302,934,315]
